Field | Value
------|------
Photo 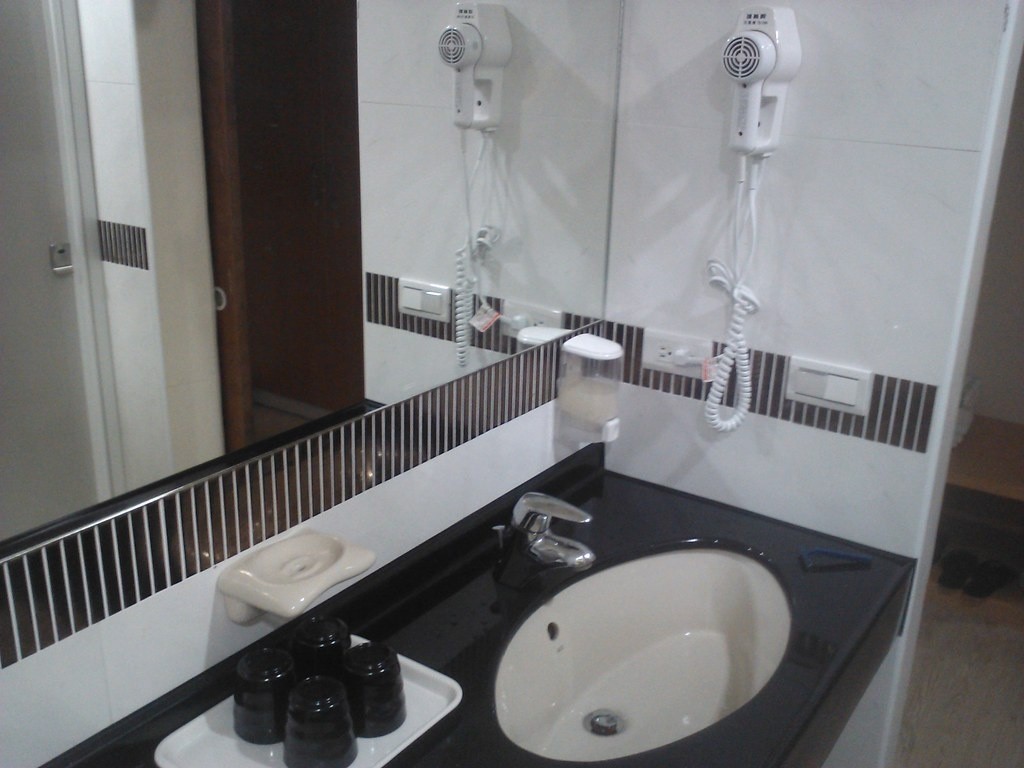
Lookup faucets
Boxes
[491,490,597,591]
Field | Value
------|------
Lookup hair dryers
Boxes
[719,28,778,156]
[436,22,483,130]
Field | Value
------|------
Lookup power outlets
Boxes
[642,329,715,379]
[500,299,563,338]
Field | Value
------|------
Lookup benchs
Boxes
[933,405,1024,565]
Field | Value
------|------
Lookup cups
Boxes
[282,675,358,768]
[343,642,406,738]
[233,648,298,746]
[291,614,352,686]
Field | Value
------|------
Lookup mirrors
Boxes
[0,0,623,563]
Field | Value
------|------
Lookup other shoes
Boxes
[939,550,1008,598]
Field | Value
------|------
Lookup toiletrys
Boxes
[555,331,625,444]
[515,324,575,354]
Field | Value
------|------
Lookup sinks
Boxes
[491,545,793,764]
